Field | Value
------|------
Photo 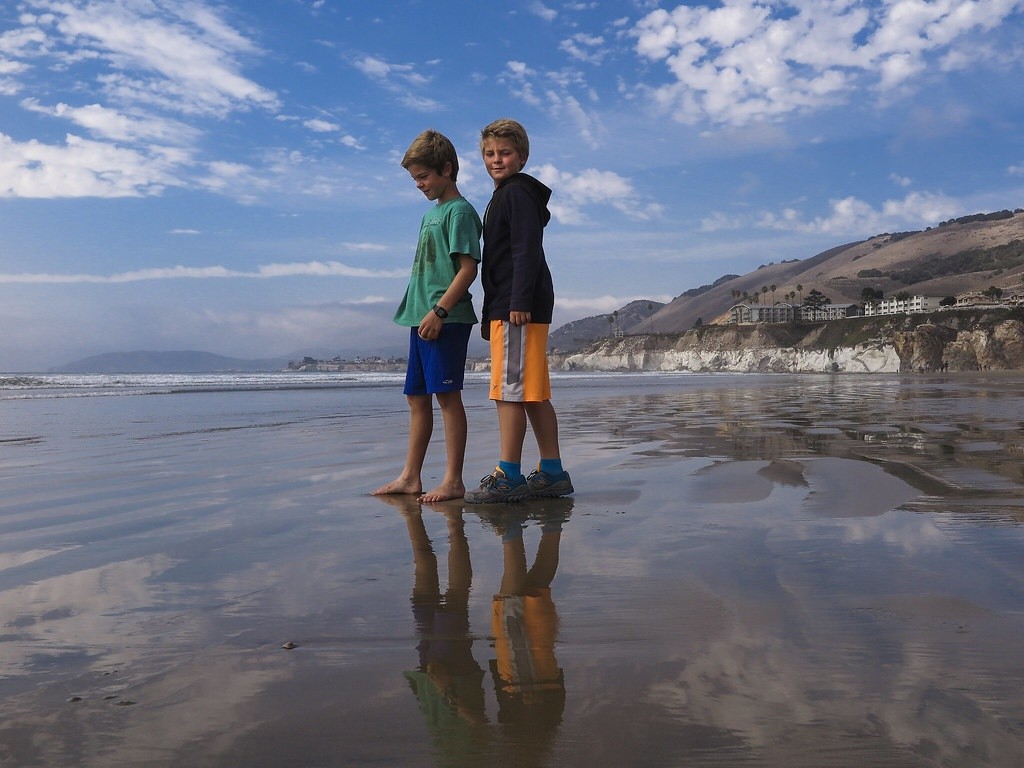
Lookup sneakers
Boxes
[465,467,529,504]
[525,469,574,498]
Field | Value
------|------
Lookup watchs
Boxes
[432,304,448,319]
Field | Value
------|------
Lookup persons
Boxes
[373,130,483,503]
[371,492,574,767]
[464,120,574,502]
